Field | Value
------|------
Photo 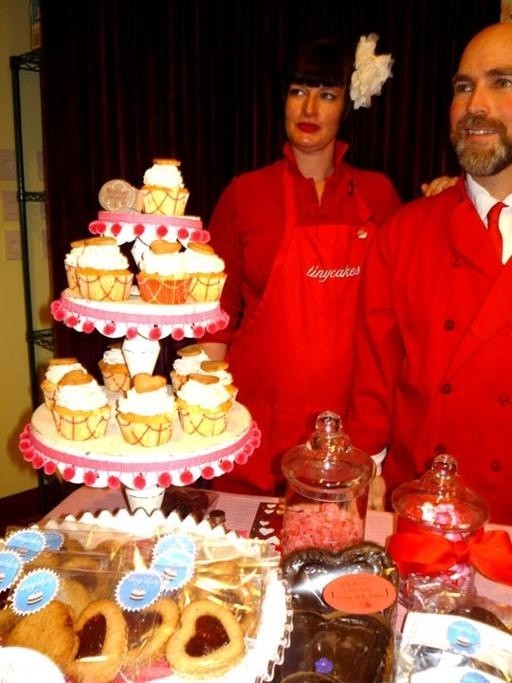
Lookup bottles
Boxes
[383,451,491,615]
[277,409,377,556]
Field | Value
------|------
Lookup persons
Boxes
[196,50,402,497]
[343,20,512,531]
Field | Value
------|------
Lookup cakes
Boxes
[17,154,262,509]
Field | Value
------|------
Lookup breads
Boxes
[1,521,266,683]
[413,605,512,683]
[276,541,401,683]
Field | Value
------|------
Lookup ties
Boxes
[485,204,508,263]
